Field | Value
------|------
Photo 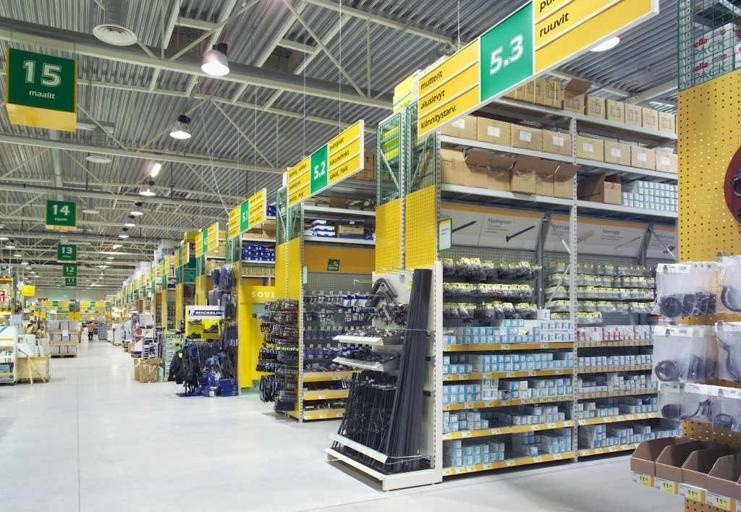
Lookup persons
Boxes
[26,316,41,334]
[86,320,95,340]
